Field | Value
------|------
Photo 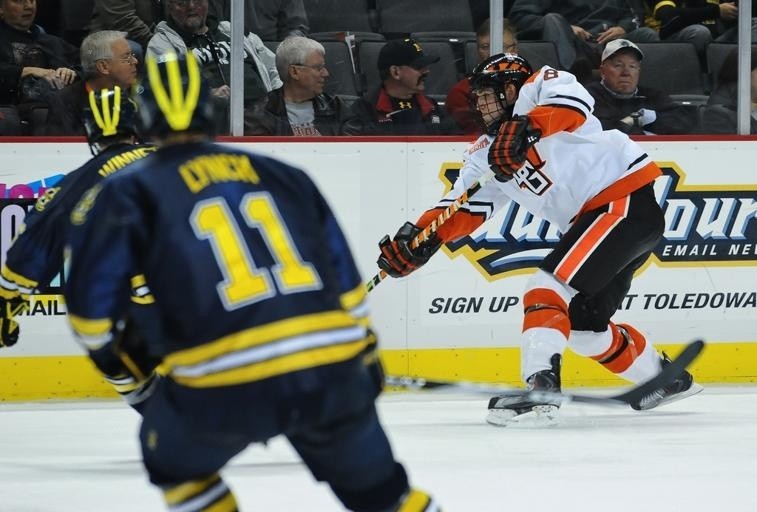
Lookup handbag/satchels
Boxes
[16,47,59,104]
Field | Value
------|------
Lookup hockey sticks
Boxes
[380,340,705,406]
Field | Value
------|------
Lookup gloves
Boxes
[486,113,532,183]
[0,317,20,349]
[376,222,444,280]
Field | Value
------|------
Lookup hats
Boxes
[600,38,644,63]
[378,37,441,71]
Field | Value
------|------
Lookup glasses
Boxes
[289,63,328,71]
[479,42,516,52]
[95,50,136,64]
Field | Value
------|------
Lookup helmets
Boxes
[469,52,535,137]
[82,87,143,147]
[138,52,216,137]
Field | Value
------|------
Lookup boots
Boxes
[487,352,563,411]
[627,350,694,412]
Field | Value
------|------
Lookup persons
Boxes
[0,86,269,452]
[445,17,519,134]
[266,36,358,136]
[345,35,457,136]
[375,51,693,413]
[0,1,282,136]
[65,54,442,510]
[512,1,756,134]
